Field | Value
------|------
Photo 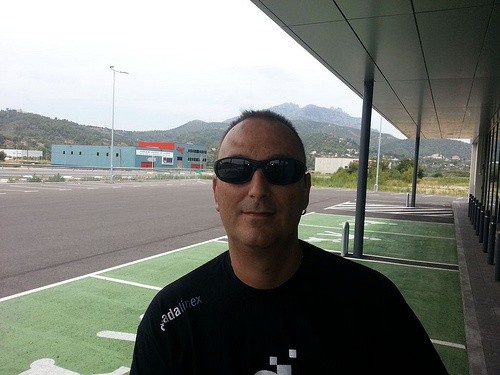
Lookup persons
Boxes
[129,110,450,375]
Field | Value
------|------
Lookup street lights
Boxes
[109,64,129,175]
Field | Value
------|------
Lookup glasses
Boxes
[214,156,308,186]
